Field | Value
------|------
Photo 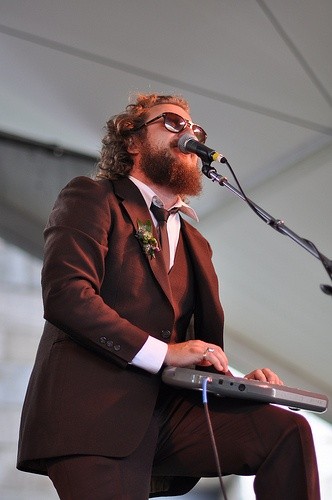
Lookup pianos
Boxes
[162,365,329,414]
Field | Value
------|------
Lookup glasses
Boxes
[131,111,208,145]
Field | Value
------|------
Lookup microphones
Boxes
[178,133,228,163]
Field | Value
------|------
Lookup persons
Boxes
[16,93,321,500]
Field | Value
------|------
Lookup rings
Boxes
[203,353,207,360]
[208,348,214,352]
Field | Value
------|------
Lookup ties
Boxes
[149,201,181,272]
